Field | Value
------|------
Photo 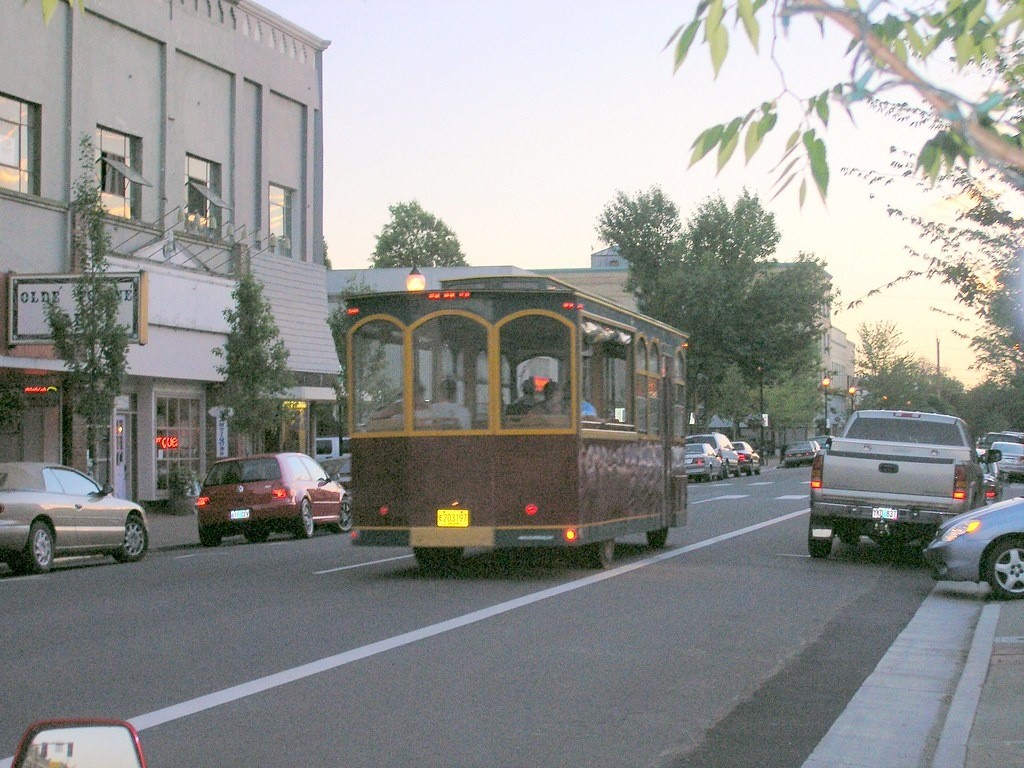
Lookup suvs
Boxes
[810,436,835,455]
[684,431,741,478]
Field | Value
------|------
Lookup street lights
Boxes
[882,395,887,410]
[757,366,765,446]
[823,377,831,436]
[849,386,856,415]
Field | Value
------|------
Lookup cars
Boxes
[685,443,724,483]
[194,452,355,549]
[0,462,151,575]
[975,448,1003,506]
[976,430,1024,449]
[730,441,761,475]
[990,442,1024,482]
[923,494,1024,601]
[810,441,821,457]
[784,441,817,468]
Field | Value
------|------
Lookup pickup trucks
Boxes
[808,410,1002,558]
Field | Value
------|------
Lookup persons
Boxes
[780,444,788,465]
[509,379,542,409]
[390,379,472,430]
[527,381,565,416]
[372,376,430,420]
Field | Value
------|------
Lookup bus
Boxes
[344,273,691,578]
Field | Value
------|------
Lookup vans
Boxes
[316,437,354,484]
